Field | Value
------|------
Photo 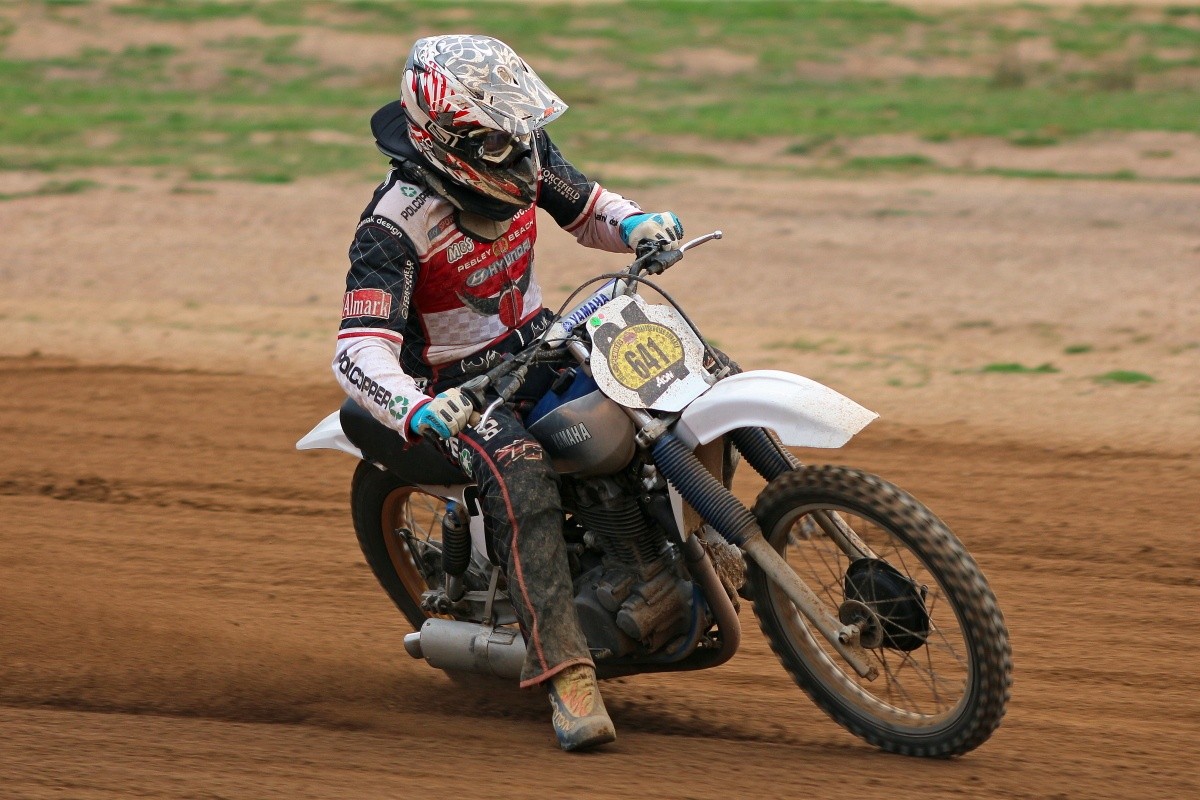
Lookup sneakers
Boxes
[548,664,618,750]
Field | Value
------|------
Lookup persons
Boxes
[331,34,684,751]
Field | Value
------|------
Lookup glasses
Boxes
[468,128,533,154]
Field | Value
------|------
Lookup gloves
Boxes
[408,388,480,441]
[619,212,682,253]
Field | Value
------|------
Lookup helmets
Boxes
[400,34,568,210]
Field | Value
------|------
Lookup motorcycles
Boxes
[295,225,1013,762]
